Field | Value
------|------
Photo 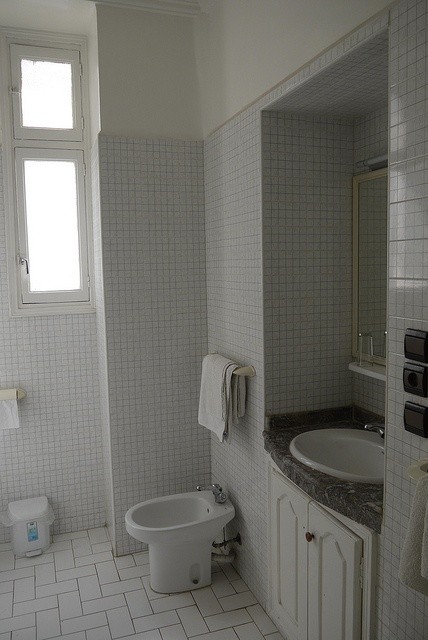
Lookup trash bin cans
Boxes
[8,496,51,558]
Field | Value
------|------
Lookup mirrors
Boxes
[350,168,388,366]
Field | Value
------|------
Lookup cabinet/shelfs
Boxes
[265,470,376,640]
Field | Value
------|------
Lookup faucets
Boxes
[364,420,385,436]
[196,484,231,502]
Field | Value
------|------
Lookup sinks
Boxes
[124,489,234,595]
[289,428,385,485]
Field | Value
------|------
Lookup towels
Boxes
[398,475,427,597]
[197,354,246,444]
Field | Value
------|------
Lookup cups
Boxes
[357,331,373,368]
